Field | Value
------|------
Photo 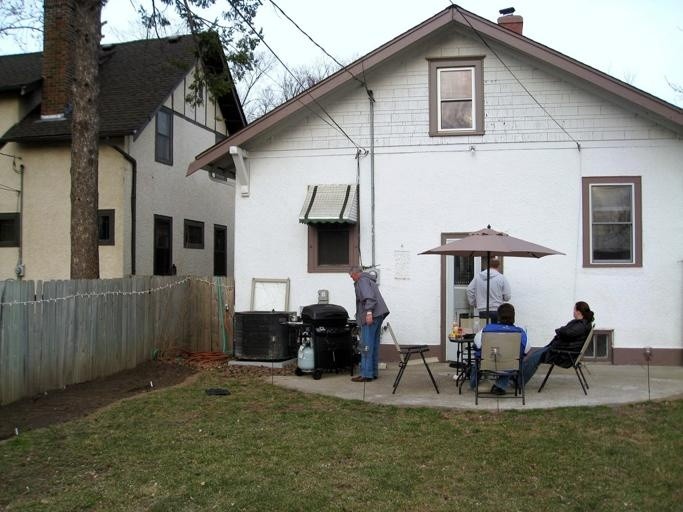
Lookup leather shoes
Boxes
[352,376,378,382]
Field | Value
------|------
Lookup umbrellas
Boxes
[416,225,567,380]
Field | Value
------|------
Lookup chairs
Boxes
[457,318,491,378]
[473,332,525,405]
[387,322,440,394]
[538,323,596,395]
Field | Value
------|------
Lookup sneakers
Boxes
[491,384,506,395]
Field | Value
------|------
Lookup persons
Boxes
[466,259,511,326]
[508,302,595,389]
[469,303,527,394]
[348,263,390,383]
